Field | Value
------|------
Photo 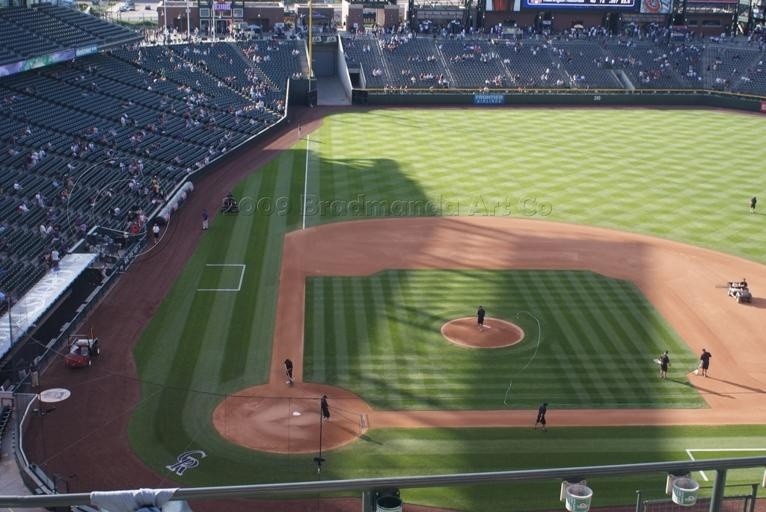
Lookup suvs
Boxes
[726,279,753,303]
[63,331,101,370]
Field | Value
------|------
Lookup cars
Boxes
[274,24,286,35]
[241,26,264,40]
[144,4,149,8]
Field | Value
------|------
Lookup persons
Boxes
[534,401,550,431]
[342,2,766,96]
[477,304,487,331]
[320,393,331,422]
[750,195,757,213]
[740,278,747,288]
[700,348,712,377]
[659,350,672,378]
[0,25,305,386]
